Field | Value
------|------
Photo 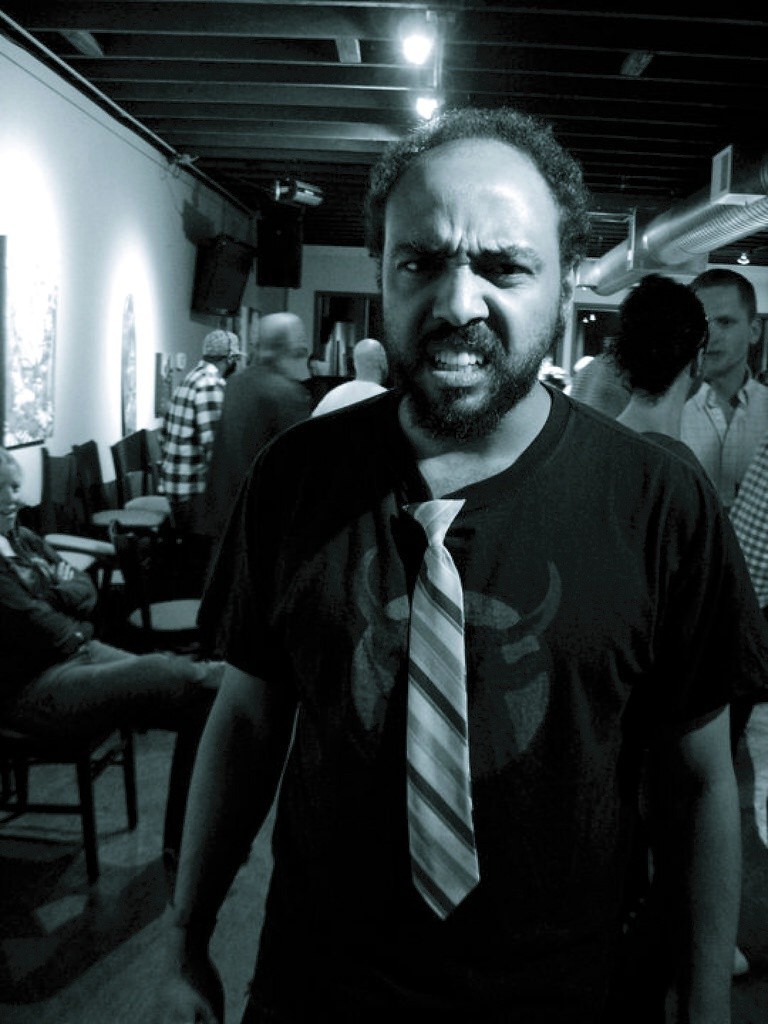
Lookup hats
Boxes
[204,330,241,357]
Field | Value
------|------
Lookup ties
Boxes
[401,500,482,919]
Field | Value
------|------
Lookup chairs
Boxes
[0,430,201,883]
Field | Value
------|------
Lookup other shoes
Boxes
[171,641,223,661]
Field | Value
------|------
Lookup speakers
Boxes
[254,217,303,288]
[191,233,256,317]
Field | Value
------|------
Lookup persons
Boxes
[0,450,231,882]
[158,108,768,1024]
